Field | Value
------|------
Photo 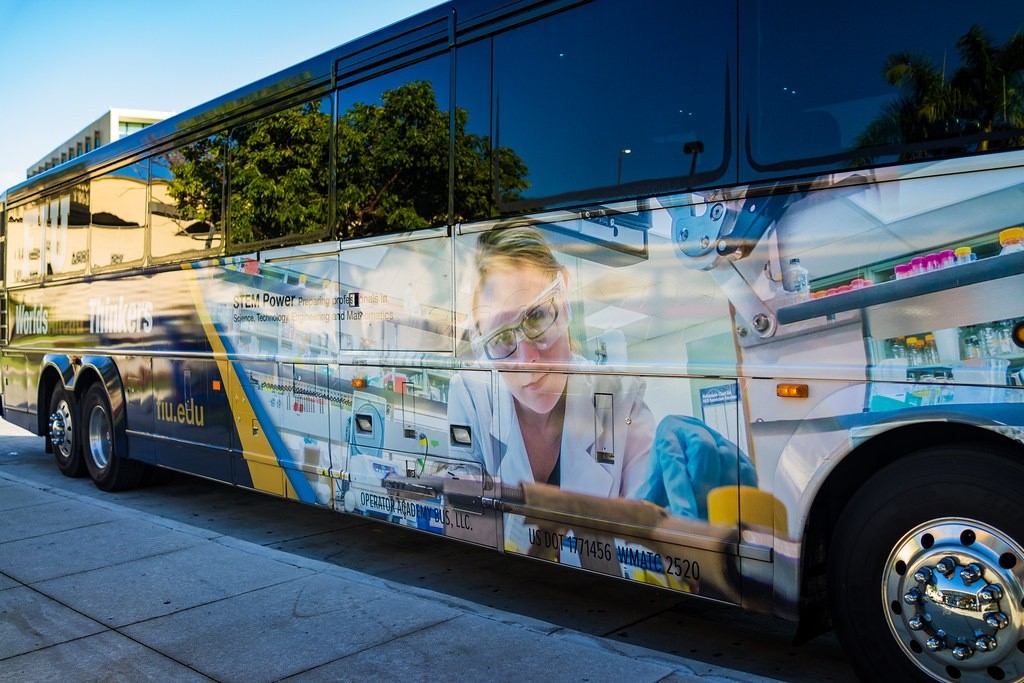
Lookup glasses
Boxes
[483,293,559,360]
[470,272,573,372]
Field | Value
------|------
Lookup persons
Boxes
[448,216,759,593]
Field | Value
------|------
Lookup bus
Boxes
[1,2,1023,681]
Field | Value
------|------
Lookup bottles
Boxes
[787,225,1023,410]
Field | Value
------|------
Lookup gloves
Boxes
[629,412,757,523]
